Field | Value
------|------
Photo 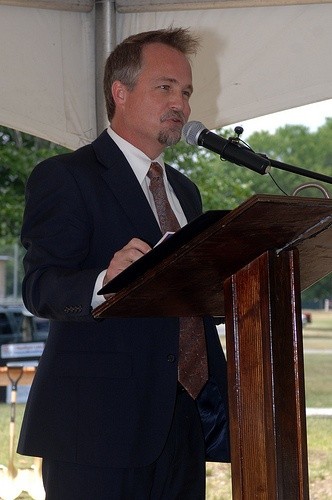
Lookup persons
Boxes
[14,25,234,500]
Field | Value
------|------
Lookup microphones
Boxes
[180,120,271,178]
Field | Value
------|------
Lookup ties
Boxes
[147,161,207,400]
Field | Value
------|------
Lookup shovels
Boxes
[0,362,25,499]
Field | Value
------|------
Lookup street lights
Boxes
[12,242,21,298]
